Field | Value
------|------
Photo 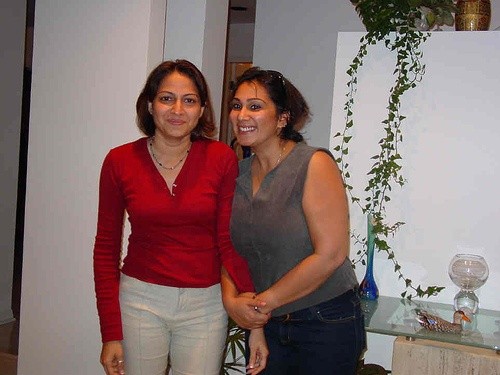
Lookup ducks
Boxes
[415,309,472,335]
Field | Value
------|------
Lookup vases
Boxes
[408,6,434,30]
[358,214,381,301]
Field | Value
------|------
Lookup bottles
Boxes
[358,214,379,300]
[359,301,379,327]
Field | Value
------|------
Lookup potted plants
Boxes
[336,1,456,234]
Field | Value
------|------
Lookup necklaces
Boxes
[150,135,192,169]
[254,138,288,187]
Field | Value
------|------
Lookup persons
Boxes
[94,60,271,375]
[221,68,367,375]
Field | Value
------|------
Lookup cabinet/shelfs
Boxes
[359,283,500,375]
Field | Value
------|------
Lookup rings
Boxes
[116,359,125,363]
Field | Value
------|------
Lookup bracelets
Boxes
[253,294,268,315]
